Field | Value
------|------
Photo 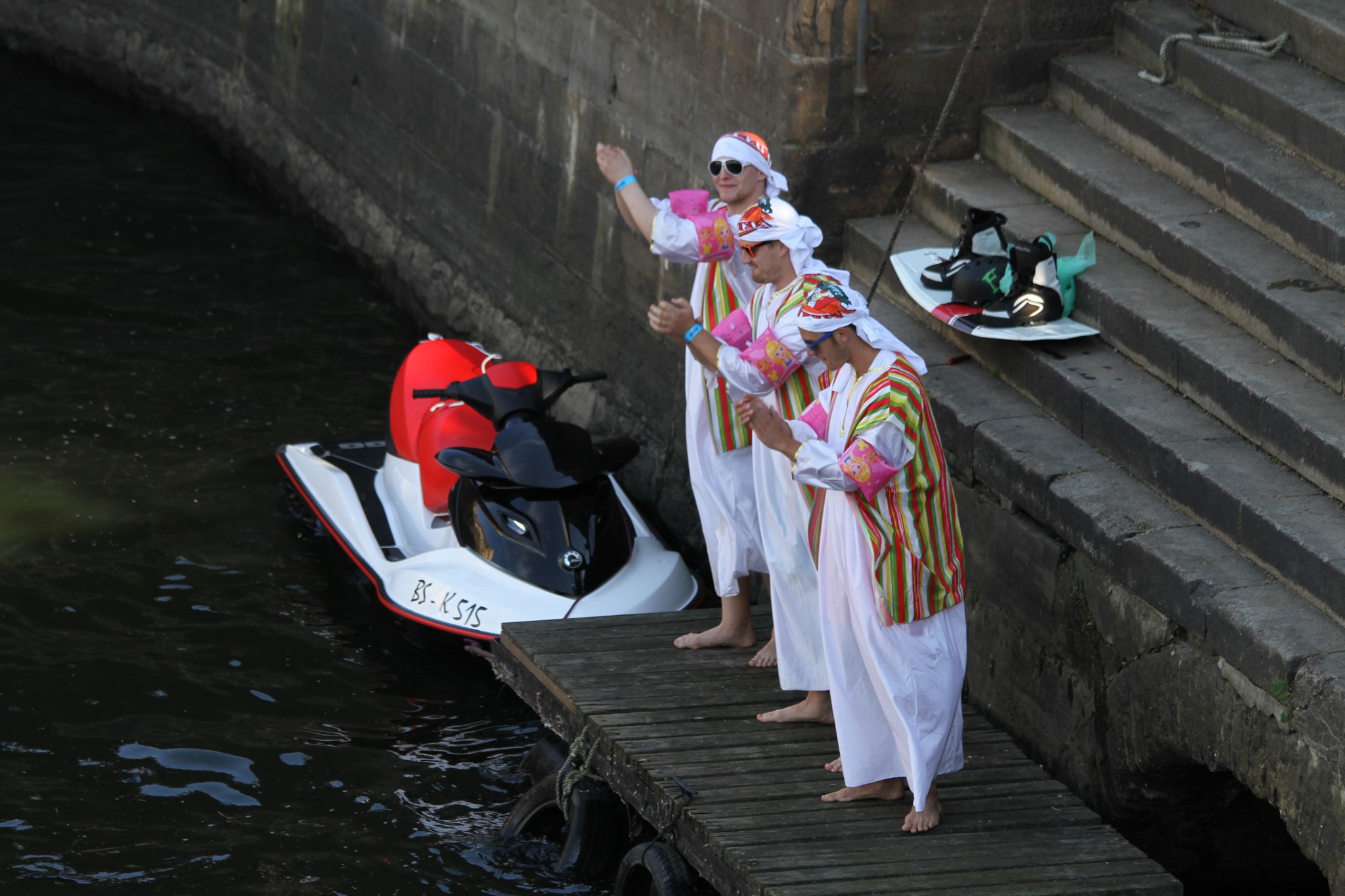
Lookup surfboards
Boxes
[888,247,1100,342]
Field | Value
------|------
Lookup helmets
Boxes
[950,256,1008,307]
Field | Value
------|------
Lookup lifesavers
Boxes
[500,767,623,876]
[616,840,698,896]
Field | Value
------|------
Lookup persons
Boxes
[648,195,851,721]
[596,131,790,668]
[728,280,969,833]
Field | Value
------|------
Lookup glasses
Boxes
[803,331,835,354]
[737,239,777,257]
[707,159,752,178]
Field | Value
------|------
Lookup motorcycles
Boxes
[277,328,698,637]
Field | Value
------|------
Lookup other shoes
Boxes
[980,239,1064,326]
[921,206,1007,287]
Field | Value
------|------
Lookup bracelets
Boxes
[683,322,703,342]
[615,175,636,192]
